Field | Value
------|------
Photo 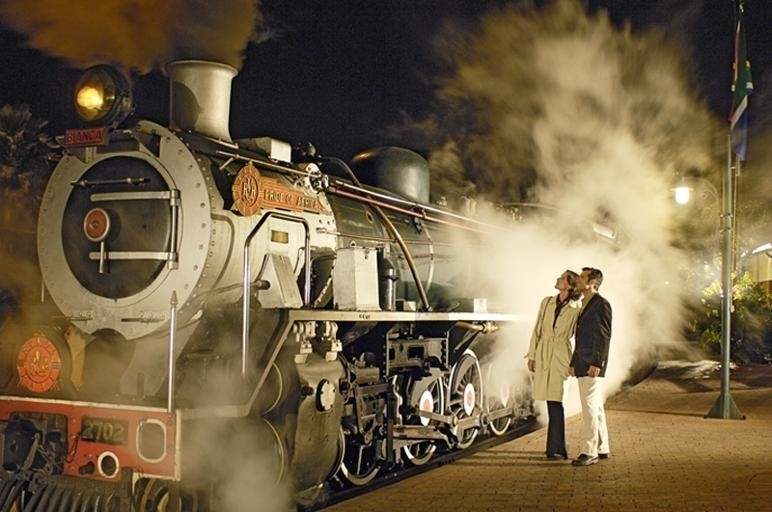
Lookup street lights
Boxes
[666,172,752,421]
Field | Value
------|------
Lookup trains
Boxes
[0,34,689,511]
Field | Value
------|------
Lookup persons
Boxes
[522,269,582,459]
[568,267,613,467]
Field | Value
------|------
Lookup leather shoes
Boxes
[547,454,563,459]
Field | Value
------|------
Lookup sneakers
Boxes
[572,453,599,466]
[597,453,608,459]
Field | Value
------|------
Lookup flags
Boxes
[725,0,755,163]
